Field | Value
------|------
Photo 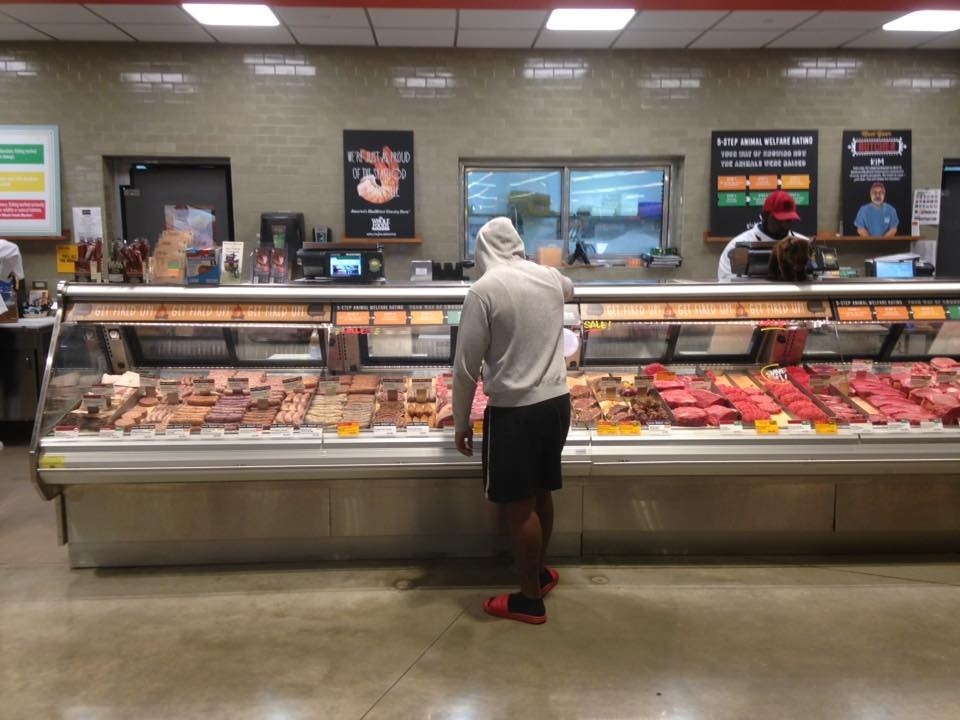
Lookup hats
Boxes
[762,190,800,221]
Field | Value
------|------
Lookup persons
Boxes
[854,183,899,239]
[717,189,812,285]
[452,215,575,626]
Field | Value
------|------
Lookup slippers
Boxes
[541,568,559,597]
[482,592,547,624]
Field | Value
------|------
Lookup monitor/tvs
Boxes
[324,251,367,280]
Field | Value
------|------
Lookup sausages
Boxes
[144,375,436,427]
